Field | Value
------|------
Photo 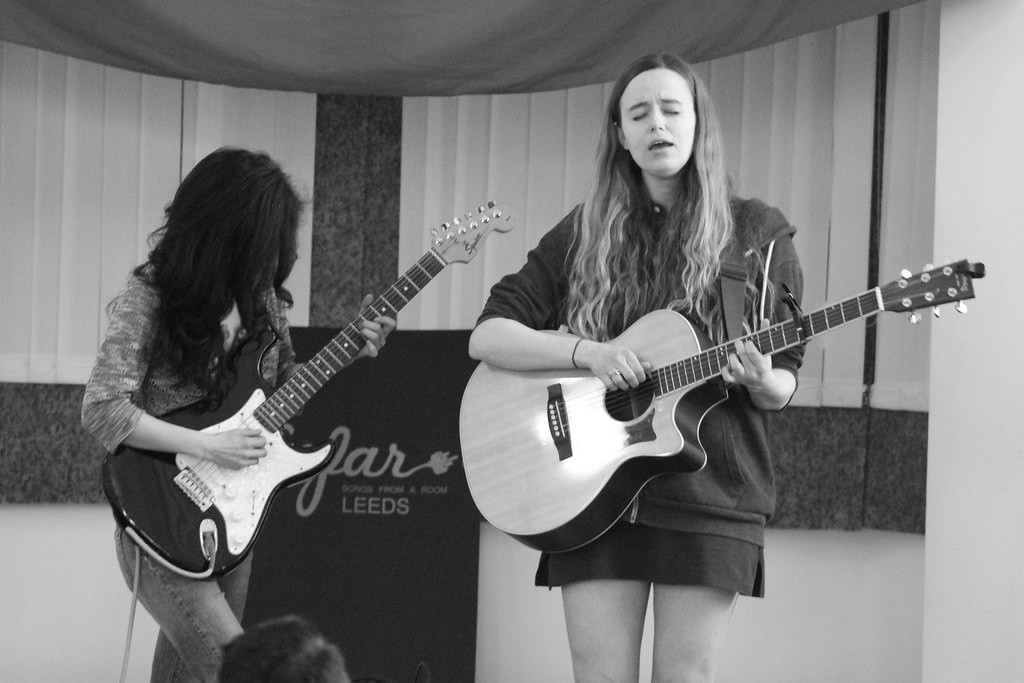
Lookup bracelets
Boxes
[571,338,585,369]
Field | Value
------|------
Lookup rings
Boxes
[609,369,620,376]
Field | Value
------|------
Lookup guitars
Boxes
[458,257,986,553]
[100,197,516,581]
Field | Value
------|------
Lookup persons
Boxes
[76,145,396,682]
[457,52,809,683]
[209,618,350,683]
